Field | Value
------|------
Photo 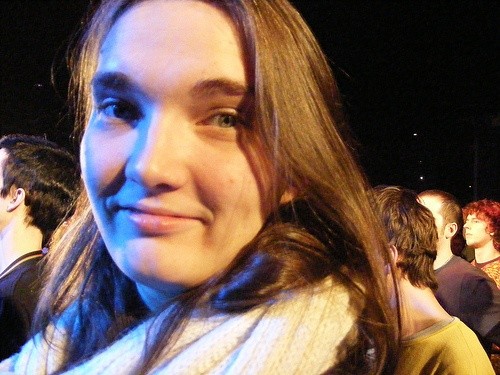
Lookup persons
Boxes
[0,0,411,375]
[0,132,500,375]
